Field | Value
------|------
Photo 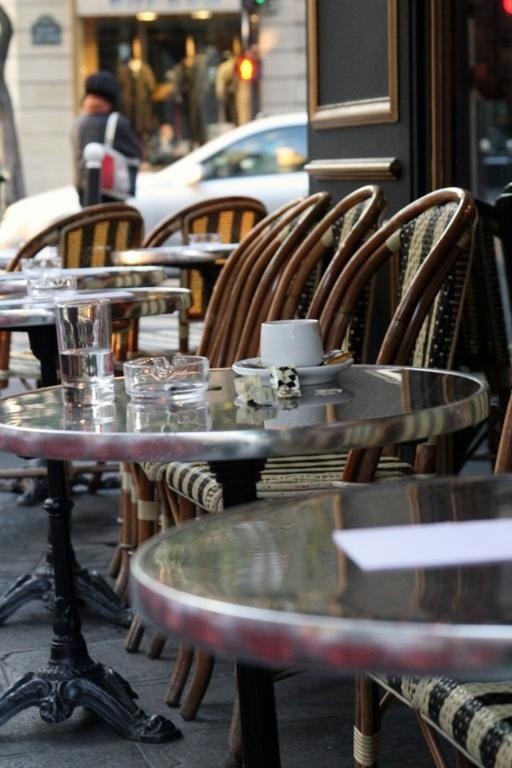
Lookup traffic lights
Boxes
[243,0,270,10]
[236,53,260,85]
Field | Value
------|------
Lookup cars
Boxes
[1,111,309,277]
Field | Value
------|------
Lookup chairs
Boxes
[1,198,145,390]
[353,383,511,767]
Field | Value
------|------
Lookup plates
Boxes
[231,351,353,388]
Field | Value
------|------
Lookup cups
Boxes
[256,319,326,371]
[23,256,61,303]
[59,297,115,408]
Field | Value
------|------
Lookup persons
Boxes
[272,141,307,172]
[119,33,253,151]
[72,72,141,205]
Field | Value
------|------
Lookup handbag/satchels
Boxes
[83,112,139,202]
